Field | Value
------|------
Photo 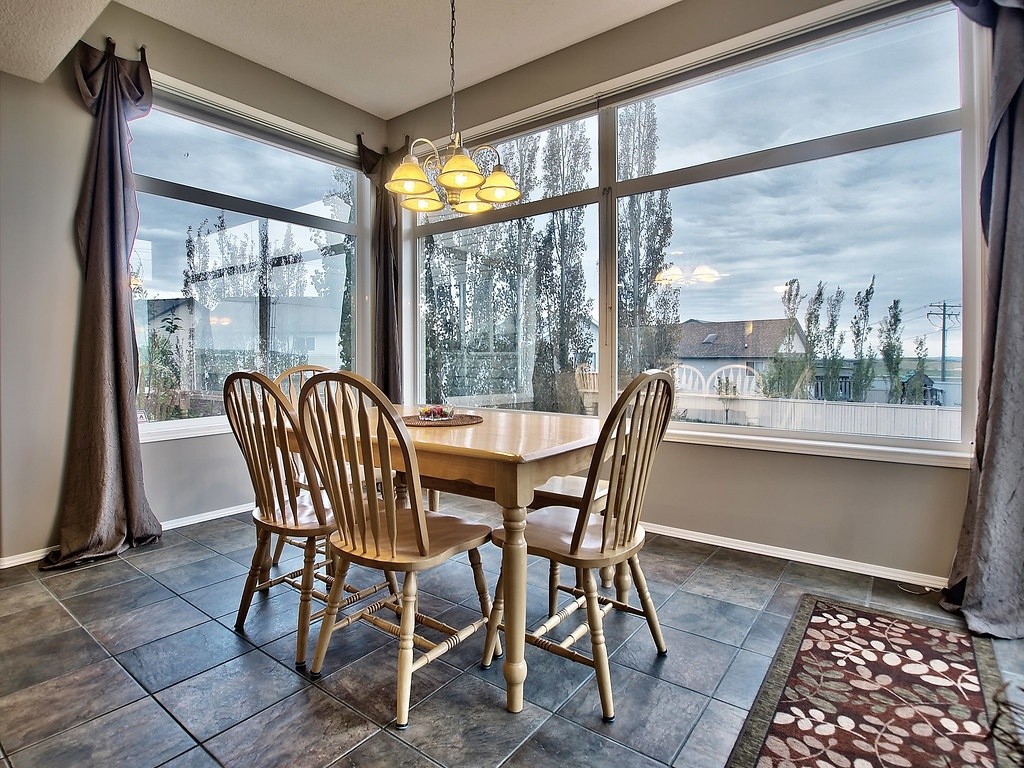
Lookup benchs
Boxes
[403,473,612,610]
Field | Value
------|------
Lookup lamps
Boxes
[384,0,523,214]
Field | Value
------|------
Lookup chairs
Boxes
[223,365,503,730]
[482,369,675,722]
[652,364,706,395]
[705,364,762,395]
[574,364,595,393]
[791,367,811,399]
[135,364,180,422]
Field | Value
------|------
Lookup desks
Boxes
[245,405,641,713]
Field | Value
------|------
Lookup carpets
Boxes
[723,592,1024,768]
